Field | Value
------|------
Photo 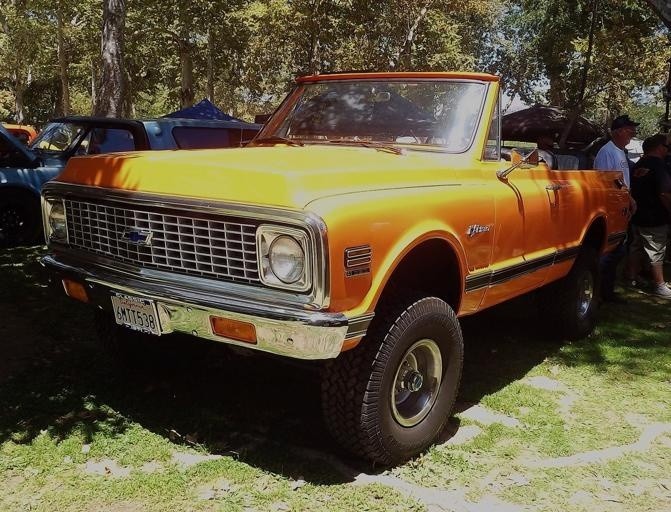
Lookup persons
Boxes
[591,115,671,298]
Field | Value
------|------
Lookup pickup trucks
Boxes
[41,70,635,465]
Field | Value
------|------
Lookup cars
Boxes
[0,113,264,240]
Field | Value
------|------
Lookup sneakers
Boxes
[651,282,671,297]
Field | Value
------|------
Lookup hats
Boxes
[611,114,640,128]
